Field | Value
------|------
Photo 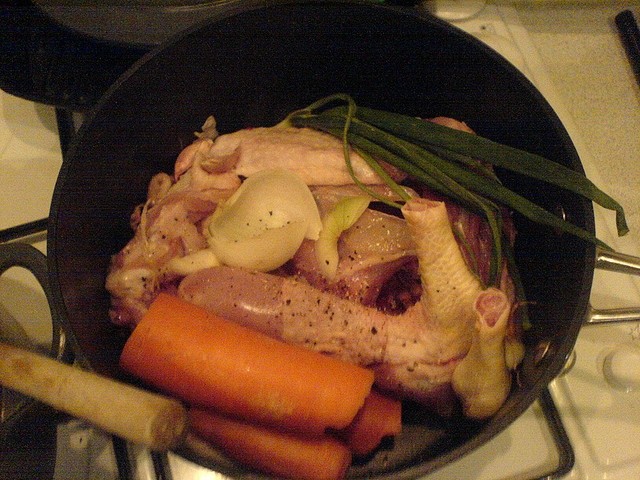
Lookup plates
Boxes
[35,0,281,47]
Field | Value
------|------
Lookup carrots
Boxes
[340,393,402,456]
[118,294,375,436]
[184,399,350,479]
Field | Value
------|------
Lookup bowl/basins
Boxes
[45,0,598,480]
